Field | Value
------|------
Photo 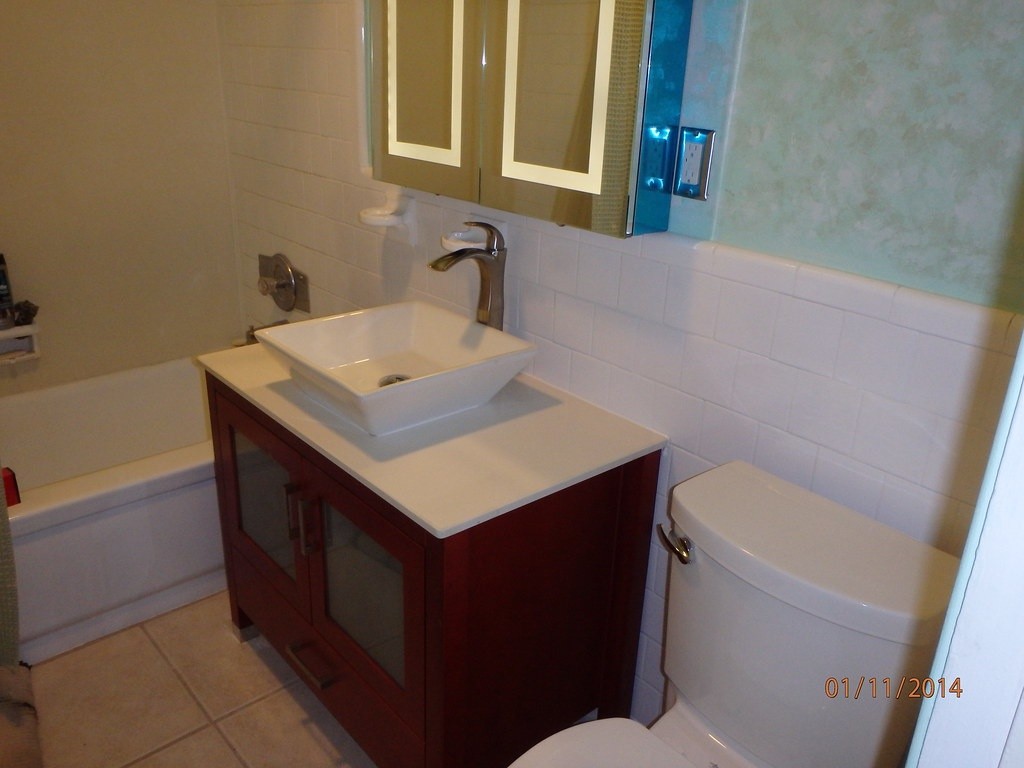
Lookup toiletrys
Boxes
[0,252,14,323]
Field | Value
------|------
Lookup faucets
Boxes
[427,221,508,332]
[233,319,288,350]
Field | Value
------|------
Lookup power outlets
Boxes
[674,126,714,200]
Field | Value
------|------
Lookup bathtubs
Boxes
[0,354,228,669]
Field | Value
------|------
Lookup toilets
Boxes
[504,459,961,768]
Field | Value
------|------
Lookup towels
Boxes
[1,472,21,673]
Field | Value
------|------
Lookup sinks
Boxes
[252,299,540,437]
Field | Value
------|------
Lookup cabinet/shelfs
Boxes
[363,1,648,239]
[203,374,664,768]
